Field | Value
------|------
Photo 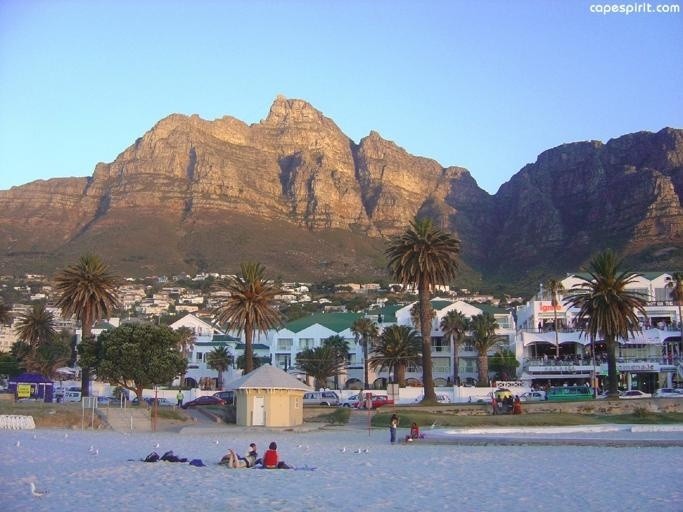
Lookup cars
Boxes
[409,392,450,404]
[653,387,683,398]
[339,394,365,408]
[596,389,623,400]
[51,385,235,407]
[518,391,545,401]
[618,389,652,399]
[468,391,494,404]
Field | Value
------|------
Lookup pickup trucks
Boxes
[353,394,394,409]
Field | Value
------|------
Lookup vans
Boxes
[302,391,339,406]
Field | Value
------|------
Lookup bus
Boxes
[548,385,593,401]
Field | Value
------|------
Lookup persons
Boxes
[527,352,593,397]
[176,389,183,408]
[389,413,399,444]
[535,318,679,333]
[258,441,280,468]
[226,447,257,469]
[493,393,522,414]
[356,388,365,409]
[244,443,263,465]
[409,422,420,440]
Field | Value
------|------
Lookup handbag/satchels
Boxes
[145,451,159,461]
